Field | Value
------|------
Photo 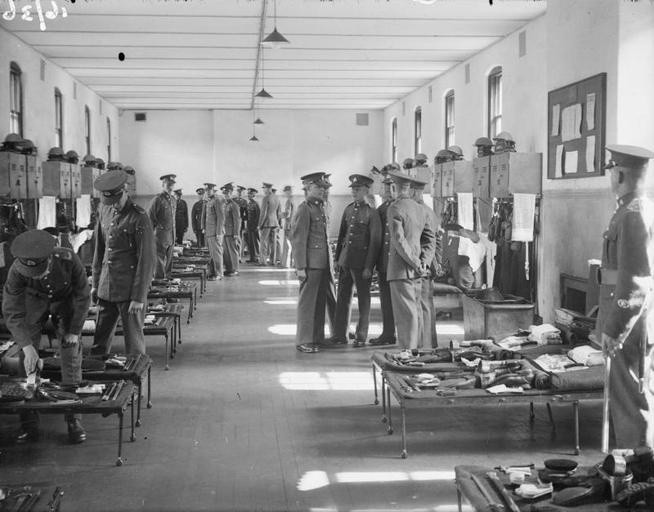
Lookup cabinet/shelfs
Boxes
[0,151,136,199]
[369,154,542,197]
[462,292,536,339]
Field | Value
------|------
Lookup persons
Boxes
[1,229,91,444]
[593,144,654,451]
[326,173,443,358]
[91,170,156,402]
[147,175,178,277]
[290,172,356,352]
[174,183,294,280]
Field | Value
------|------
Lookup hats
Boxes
[160,175,182,195]
[382,172,429,189]
[348,175,373,188]
[94,170,127,205]
[301,172,332,190]
[600,144,654,169]
[11,230,55,277]
[196,184,258,194]
[262,183,276,192]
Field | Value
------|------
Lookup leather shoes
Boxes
[297,332,396,353]
[11,422,39,443]
[68,418,87,442]
[208,271,239,281]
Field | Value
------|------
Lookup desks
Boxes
[560,270,590,314]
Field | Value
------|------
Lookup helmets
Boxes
[0,134,37,156]
[107,162,135,175]
[475,132,516,157]
[47,147,78,164]
[403,154,427,169]
[84,155,104,169]
[434,146,462,164]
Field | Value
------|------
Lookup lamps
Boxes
[250,0,290,141]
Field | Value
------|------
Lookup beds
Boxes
[372,327,653,511]
[2,233,212,511]
